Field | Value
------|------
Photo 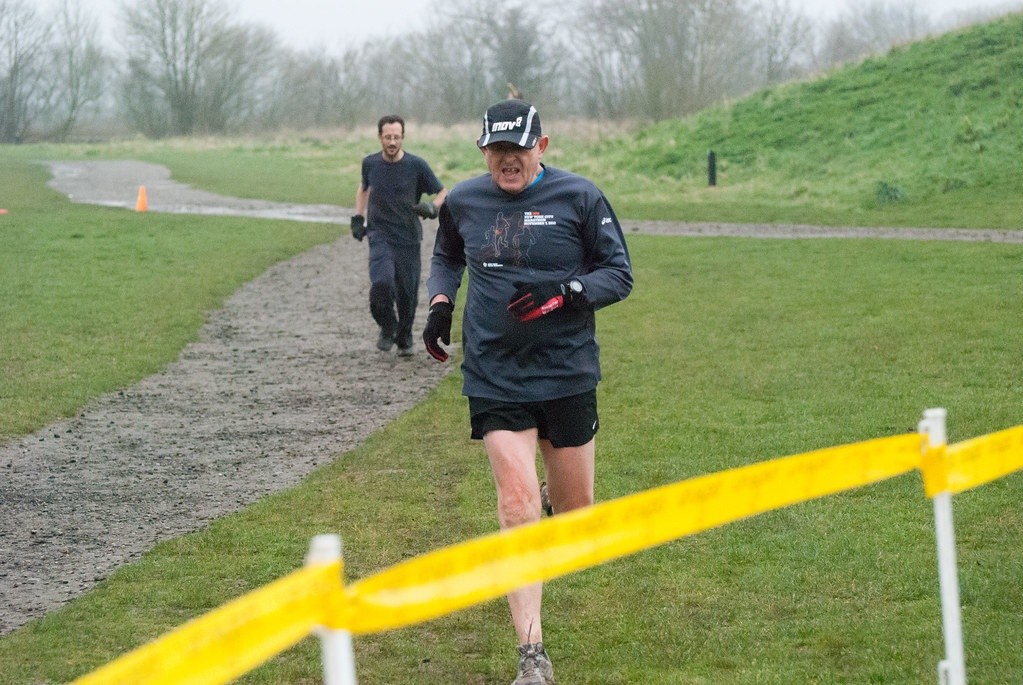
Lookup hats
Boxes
[478,100,541,149]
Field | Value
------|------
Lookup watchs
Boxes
[566,280,583,303]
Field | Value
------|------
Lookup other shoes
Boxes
[377,321,399,351]
[397,334,416,355]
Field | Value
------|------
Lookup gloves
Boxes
[507,278,571,325]
[350,215,367,241]
[411,202,439,220]
[423,302,454,362]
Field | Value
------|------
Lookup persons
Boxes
[422,99,635,685]
[351,116,448,357]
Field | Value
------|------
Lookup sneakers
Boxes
[540,482,554,516]
[510,625,555,685]
